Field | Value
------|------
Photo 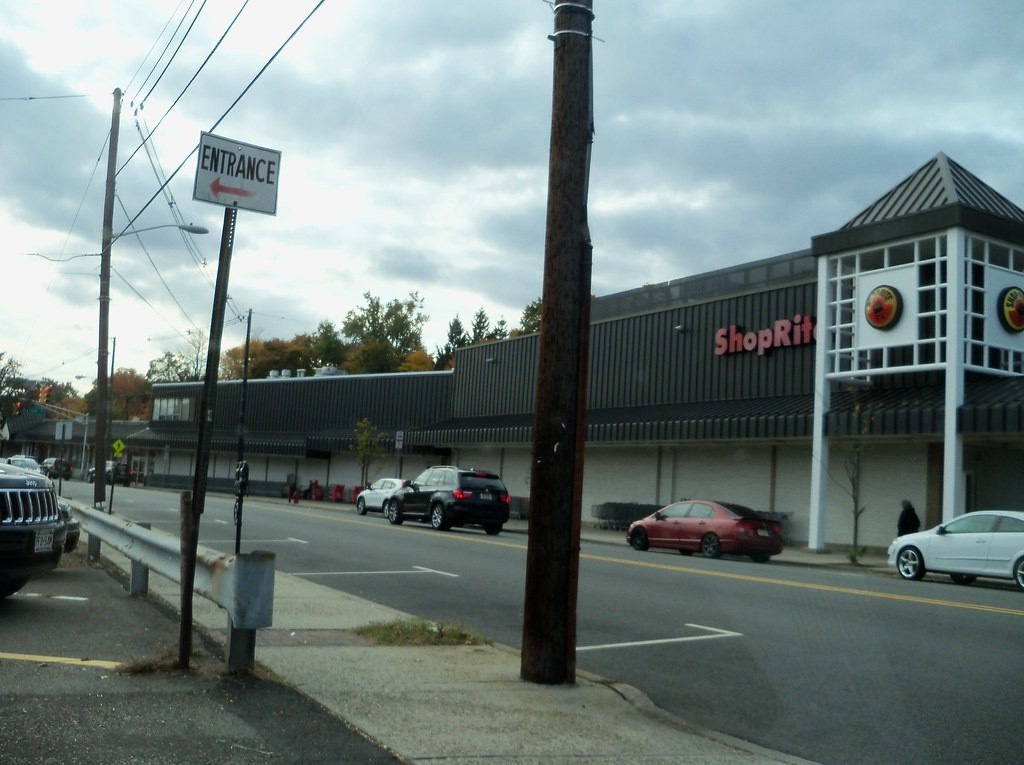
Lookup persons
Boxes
[897,499,920,538]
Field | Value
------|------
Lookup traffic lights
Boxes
[39,385,51,405]
[12,400,22,417]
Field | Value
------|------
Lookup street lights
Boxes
[94,222,210,508]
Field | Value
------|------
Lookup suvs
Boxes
[0,463,80,600]
[87,461,131,487]
[387,465,510,536]
[42,458,73,481]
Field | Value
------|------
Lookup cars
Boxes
[626,499,783,564]
[356,477,414,516]
[885,509,1024,594]
[5,457,46,476]
